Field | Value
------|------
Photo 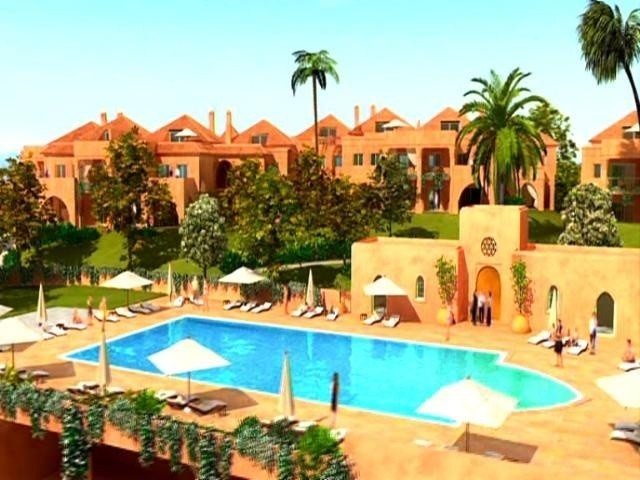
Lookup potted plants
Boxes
[433,253,458,326]
[509,260,533,334]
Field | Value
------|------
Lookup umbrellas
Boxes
[0,316,45,369]
[548,288,558,331]
[147,338,232,399]
[305,267,314,307]
[216,265,267,301]
[99,270,155,307]
[364,275,408,315]
[35,281,49,322]
[276,351,297,417]
[97,327,110,392]
[416,375,519,452]
[165,263,175,301]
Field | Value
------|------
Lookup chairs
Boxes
[64,379,127,402]
[526,328,590,356]
[289,302,340,320]
[222,299,273,313]
[37,300,161,341]
[359,308,401,328]
[265,415,348,445]
[151,389,228,417]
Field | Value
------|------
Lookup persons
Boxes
[587,311,597,355]
[329,372,339,427]
[485,291,493,327]
[72,306,83,323]
[570,328,578,347]
[551,318,565,369]
[202,278,210,312]
[280,282,291,317]
[191,275,201,311]
[86,296,94,327]
[621,338,635,363]
[476,292,486,324]
[99,296,116,318]
[566,328,572,348]
[469,292,478,326]
